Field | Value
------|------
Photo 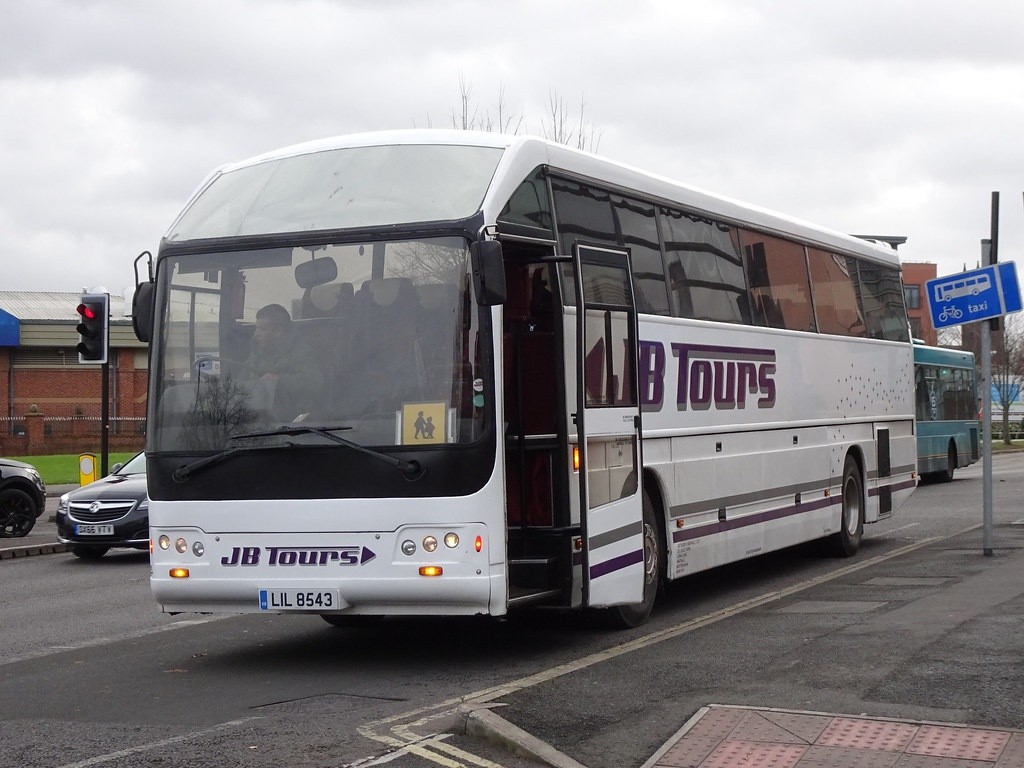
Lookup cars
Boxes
[56,450,150,560]
[0,458,48,538]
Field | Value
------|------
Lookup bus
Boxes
[132,126,920,630]
[912,338,982,483]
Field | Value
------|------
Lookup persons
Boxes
[240,304,321,421]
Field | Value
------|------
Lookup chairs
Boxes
[297,277,557,527]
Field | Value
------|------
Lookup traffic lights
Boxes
[75,293,108,364]
